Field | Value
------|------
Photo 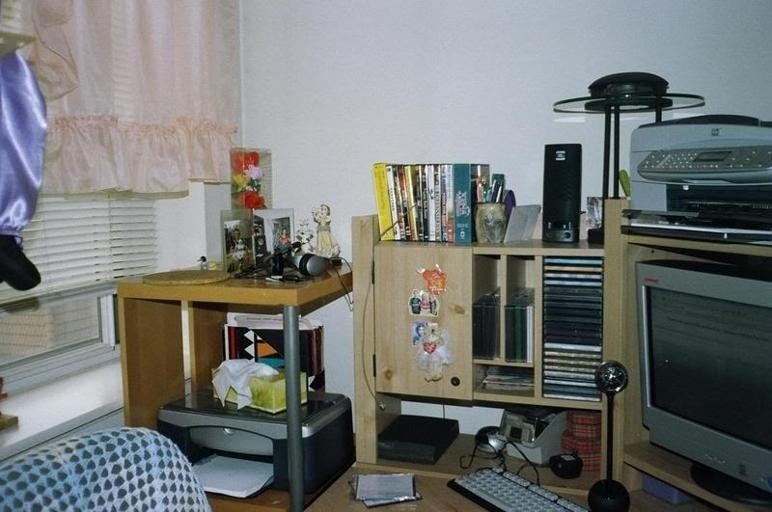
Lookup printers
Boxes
[156,392,353,502]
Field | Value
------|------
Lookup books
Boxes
[472,286,535,391]
[543,258,603,402]
[224,312,325,393]
[372,163,490,243]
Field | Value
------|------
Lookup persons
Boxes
[408,289,437,316]
[231,228,249,266]
[278,228,288,244]
[311,204,340,259]
[413,323,426,346]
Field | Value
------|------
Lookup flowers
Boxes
[231,150,265,210]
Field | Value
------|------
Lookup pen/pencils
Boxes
[477,177,503,203]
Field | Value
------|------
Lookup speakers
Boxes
[542,142,581,245]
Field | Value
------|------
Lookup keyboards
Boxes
[448,468,590,512]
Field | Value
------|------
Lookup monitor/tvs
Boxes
[634,259,772,507]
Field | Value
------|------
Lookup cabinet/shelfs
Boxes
[601,196,772,512]
[352,195,646,499]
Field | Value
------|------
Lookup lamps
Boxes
[550,71,707,243]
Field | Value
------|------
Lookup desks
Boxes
[115,262,356,509]
